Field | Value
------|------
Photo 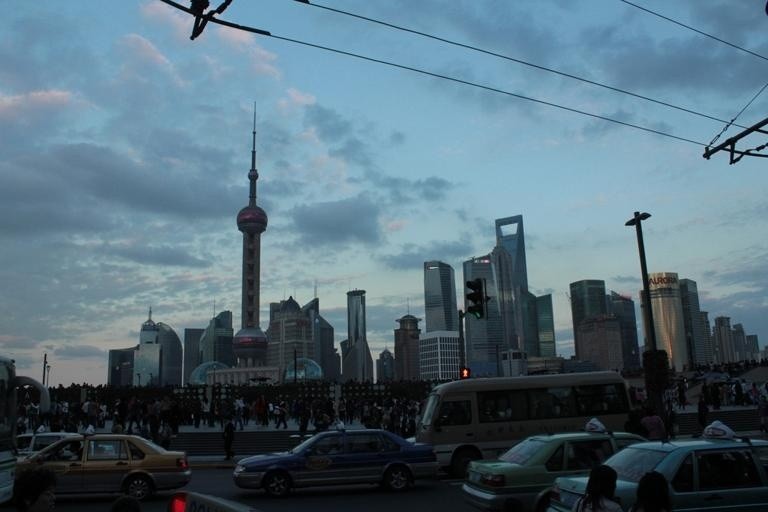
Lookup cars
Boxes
[529,422,767,511]
[232,422,440,498]
[13,434,194,512]
[460,418,650,512]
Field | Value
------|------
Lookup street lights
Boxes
[626,211,656,352]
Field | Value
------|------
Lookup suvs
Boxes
[15,432,115,463]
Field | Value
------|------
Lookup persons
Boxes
[573,465,624,512]
[15,382,179,459]
[480,399,622,421]
[0,468,56,512]
[628,471,675,512]
[616,358,766,442]
[180,376,461,439]
[112,497,141,512]
[222,416,236,459]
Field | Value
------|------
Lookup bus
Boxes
[0,356,50,504]
[416,371,634,476]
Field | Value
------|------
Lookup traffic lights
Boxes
[460,368,470,378]
[466,278,484,319]
